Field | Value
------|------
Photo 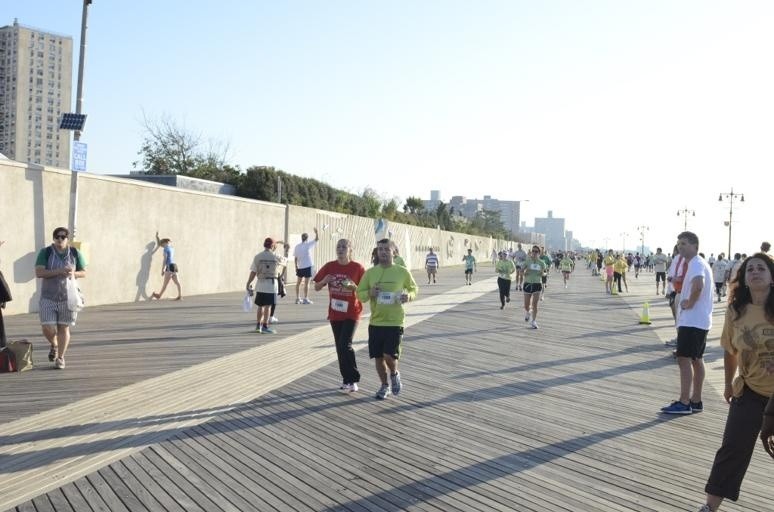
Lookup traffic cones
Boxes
[611,281,618,295]
[638,300,653,325]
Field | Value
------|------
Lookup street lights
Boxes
[676,203,697,232]
[509,198,531,252]
[636,223,651,255]
[618,231,629,252]
[717,184,746,262]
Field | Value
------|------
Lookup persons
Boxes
[293,227,320,305]
[246,237,285,334]
[258,243,292,324]
[491,243,575,328]
[311,238,365,395]
[35,227,88,370]
[584,242,774,358]
[153,231,182,301]
[355,239,417,399]
[462,249,477,285]
[659,231,714,412]
[425,247,439,284]
[0,272,13,352]
[701,253,774,512]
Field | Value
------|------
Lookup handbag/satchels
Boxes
[1,339,34,374]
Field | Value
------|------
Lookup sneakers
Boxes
[261,326,275,334]
[530,321,538,329]
[390,370,402,395]
[269,316,278,324]
[296,298,303,304]
[661,400,703,414]
[153,291,160,299]
[302,298,313,304]
[524,310,531,321]
[48,345,57,362]
[376,384,391,399]
[55,358,66,370]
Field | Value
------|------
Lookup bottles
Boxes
[251,284,254,297]
[342,280,354,288]
[396,287,408,304]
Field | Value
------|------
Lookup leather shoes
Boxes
[350,383,358,392]
